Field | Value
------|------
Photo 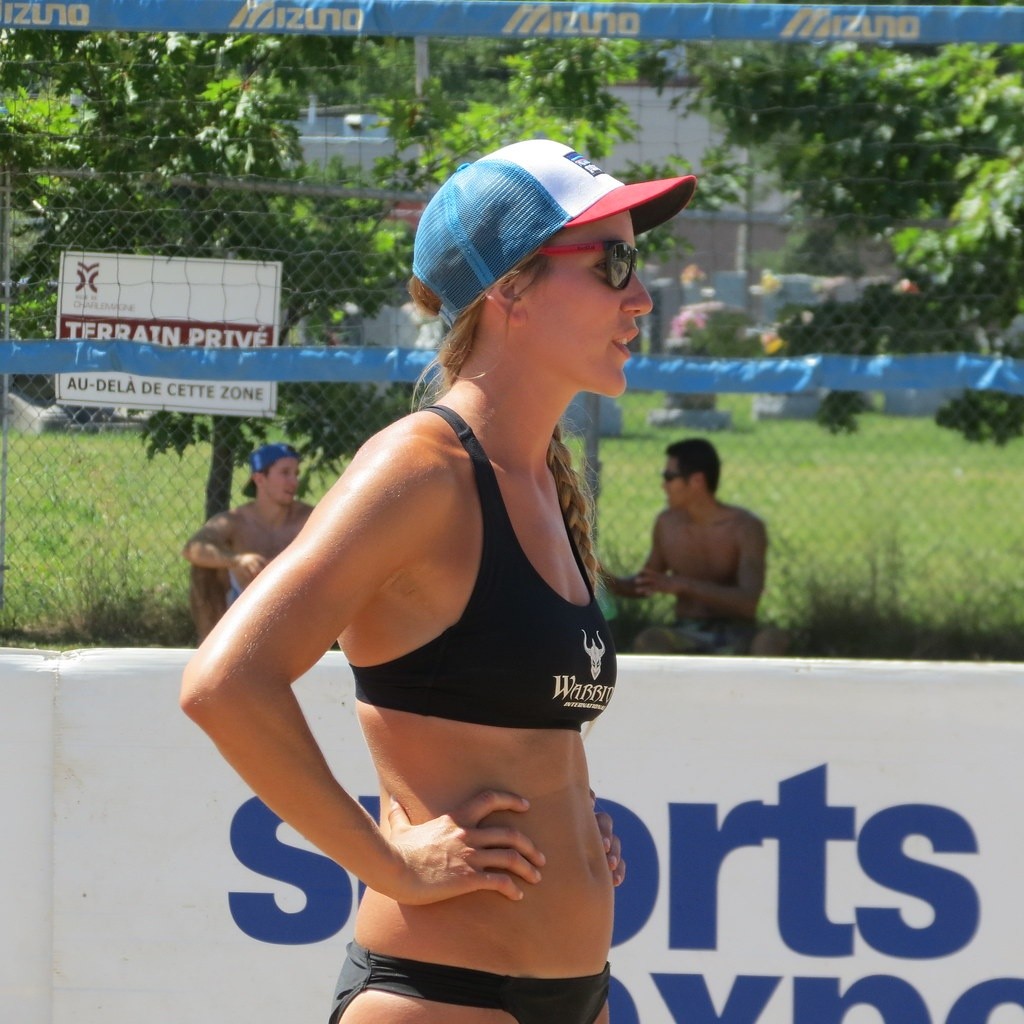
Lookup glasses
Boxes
[537,238,640,290]
[662,470,680,481]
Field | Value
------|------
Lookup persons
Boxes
[592,437,787,658]
[179,138,701,1024]
[184,442,314,657]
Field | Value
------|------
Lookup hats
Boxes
[241,443,300,498]
[413,138,699,330]
